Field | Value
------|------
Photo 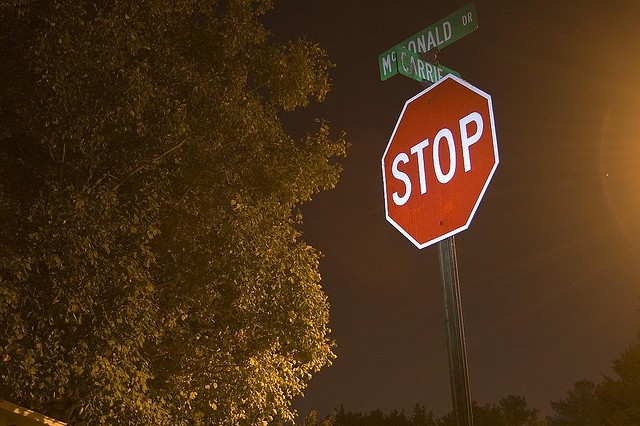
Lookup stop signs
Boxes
[380,71,500,249]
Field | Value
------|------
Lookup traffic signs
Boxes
[396,45,461,86]
[377,0,479,81]
